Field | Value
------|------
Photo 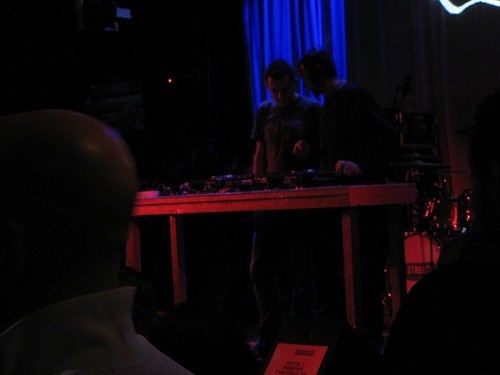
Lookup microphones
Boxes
[401,76,411,100]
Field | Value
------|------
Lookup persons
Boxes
[383,88,500,375]
[0,109,194,375]
[292,50,395,339]
[250,62,321,362]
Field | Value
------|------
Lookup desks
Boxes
[127,184,416,326]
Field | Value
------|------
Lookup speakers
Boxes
[258,318,383,375]
